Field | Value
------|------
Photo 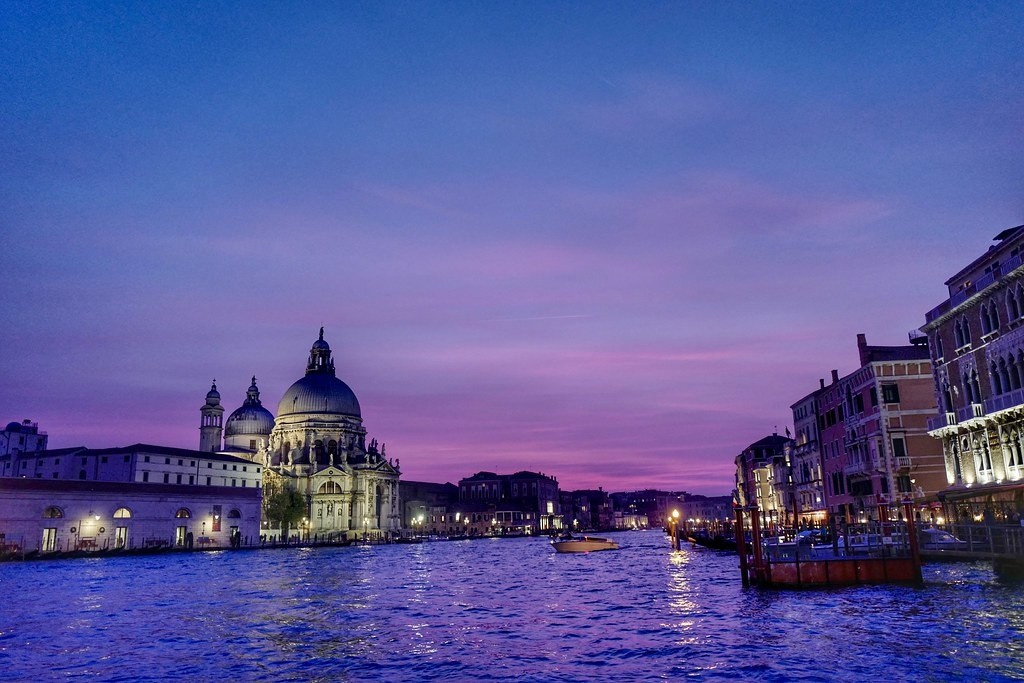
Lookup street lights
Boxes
[668,508,679,525]
[301,517,309,540]
[363,517,369,538]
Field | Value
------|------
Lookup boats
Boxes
[809,528,960,556]
[766,530,821,558]
[549,535,619,552]
